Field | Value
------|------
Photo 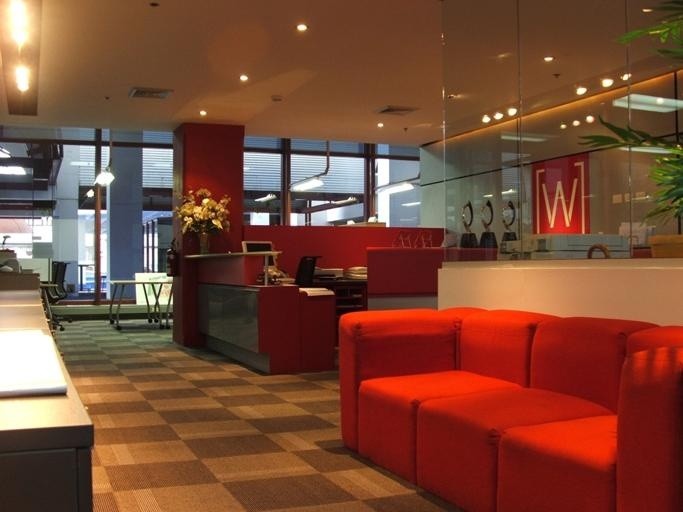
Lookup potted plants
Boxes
[577,0,683,258]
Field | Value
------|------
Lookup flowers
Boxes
[172,187,231,234]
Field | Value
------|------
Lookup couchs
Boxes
[336,307,683,512]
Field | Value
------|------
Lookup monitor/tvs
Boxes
[241,240,278,271]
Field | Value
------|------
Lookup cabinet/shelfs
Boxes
[316,280,367,348]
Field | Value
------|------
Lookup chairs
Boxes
[34,260,77,330]
[295,255,317,285]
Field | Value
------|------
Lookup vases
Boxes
[198,234,207,253]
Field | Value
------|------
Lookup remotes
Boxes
[320,276,342,280]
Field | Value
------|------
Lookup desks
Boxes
[40,284,58,333]
[107,281,172,331]
[0,272,94,512]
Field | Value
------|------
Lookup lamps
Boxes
[289,141,331,194]
[253,193,276,203]
[93,129,116,189]
[373,172,421,197]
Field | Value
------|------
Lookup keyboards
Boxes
[272,277,295,282]
